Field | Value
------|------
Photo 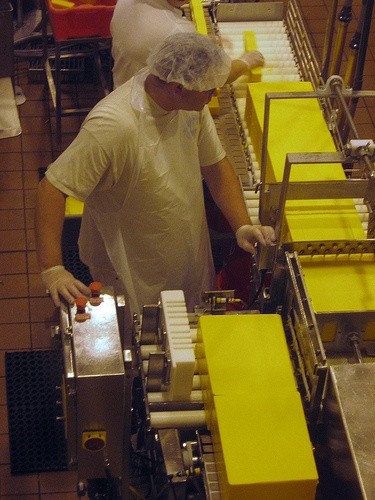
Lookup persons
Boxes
[34,32,276,308]
[109,0,265,93]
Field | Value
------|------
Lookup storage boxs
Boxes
[47,0,117,41]
